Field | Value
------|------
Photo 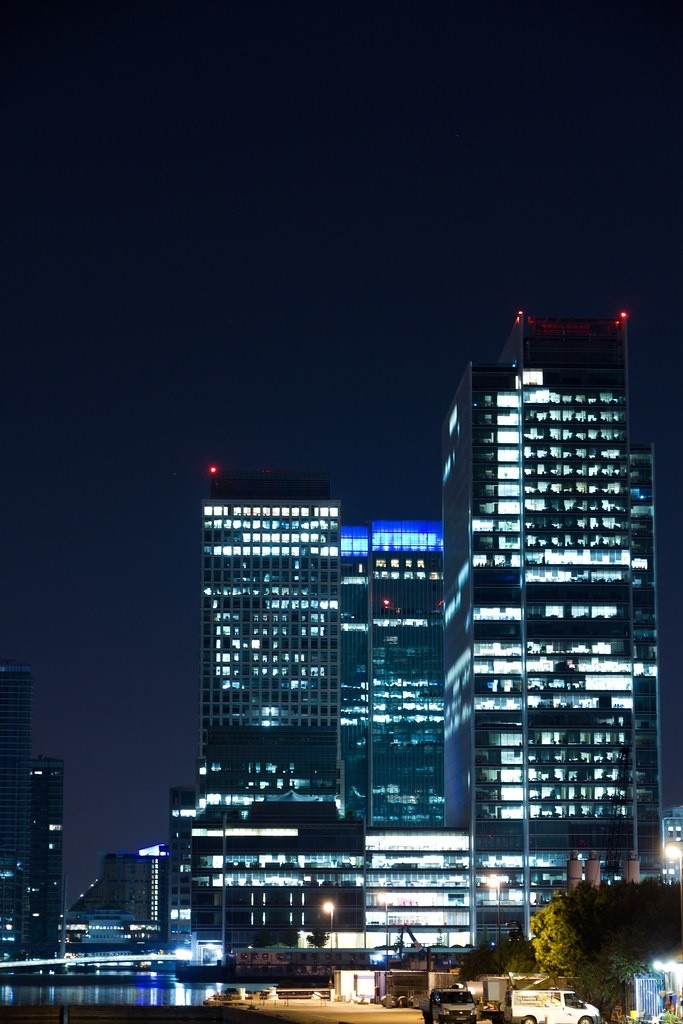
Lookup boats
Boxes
[203,988,263,1007]
[255,983,331,1000]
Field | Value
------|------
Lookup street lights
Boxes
[323,903,334,988]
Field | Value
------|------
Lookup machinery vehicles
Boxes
[395,923,452,972]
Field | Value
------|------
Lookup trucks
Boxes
[418,987,477,1024]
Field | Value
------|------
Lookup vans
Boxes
[505,989,601,1024]
